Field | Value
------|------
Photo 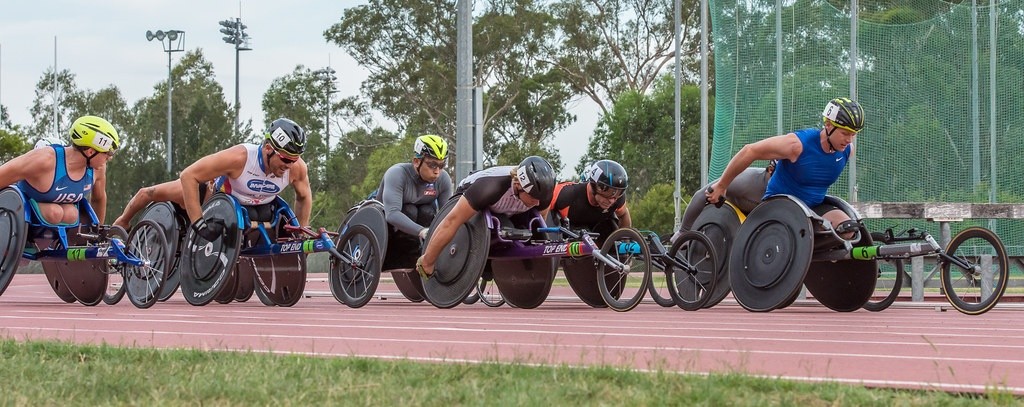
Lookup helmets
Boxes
[265,117,307,156]
[516,156,556,202]
[590,160,628,189]
[68,115,119,153]
[770,159,778,167]
[822,97,865,132]
[413,135,450,160]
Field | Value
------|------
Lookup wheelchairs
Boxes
[331,190,719,312]
[675,188,1008,315]
[0,184,168,307]
[129,191,381,309]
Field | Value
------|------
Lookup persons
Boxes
[111,118,312,242]
[415,157,556,275]
[670,95,866,246]
[0,116,119,227]
[549,159,631,231]
[375,135,453,252]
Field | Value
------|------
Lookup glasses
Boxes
[418,158,446,170]
[268,142,297,164]
[107,150,117,156]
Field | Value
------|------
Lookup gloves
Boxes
[191,217,224,242]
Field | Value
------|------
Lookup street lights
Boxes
[218,18,252,150]
[146,30,188,177]
[318,55,340,183]
